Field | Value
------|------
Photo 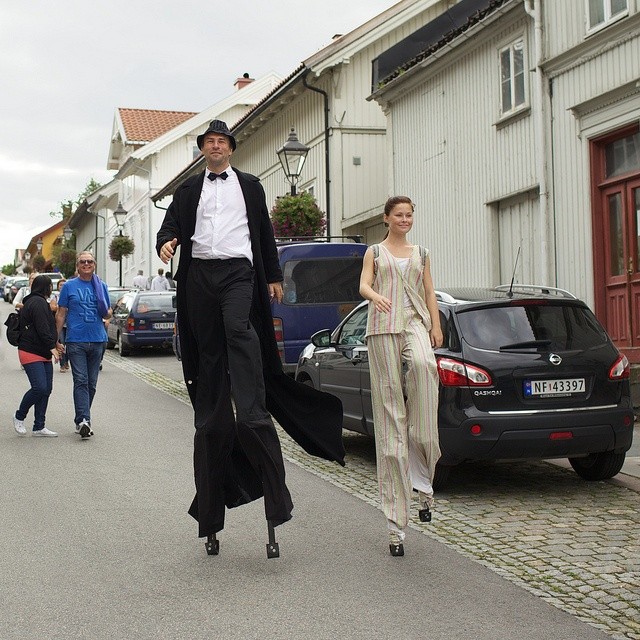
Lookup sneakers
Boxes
[75,421,94,440]
[32,428,58,437]
[13,414,27,436]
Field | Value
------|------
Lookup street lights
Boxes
[113,201,129,287]
[275,125,311,199]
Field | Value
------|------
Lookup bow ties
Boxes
[208,172,228,181]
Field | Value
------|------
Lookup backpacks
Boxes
[4,299,29,346]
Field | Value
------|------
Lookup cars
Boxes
[3,271,67,304]
[102,287,179,357]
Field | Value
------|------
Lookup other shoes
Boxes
[60,362,70,373]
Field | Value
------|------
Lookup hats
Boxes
[196,120,236,152]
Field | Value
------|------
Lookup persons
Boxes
[165,272,175,291]
[134,269,150,290]
[12,272,113,371]
[150,268,170,291]
[12,275,59,438]
[54,253,113,438]
[0,276,8,298]
[359,196,443,556]
[155,120,294,558]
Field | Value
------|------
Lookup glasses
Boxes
[80,259,95,264]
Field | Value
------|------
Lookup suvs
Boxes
[266,234,371,375]
[294,282,635,492]
